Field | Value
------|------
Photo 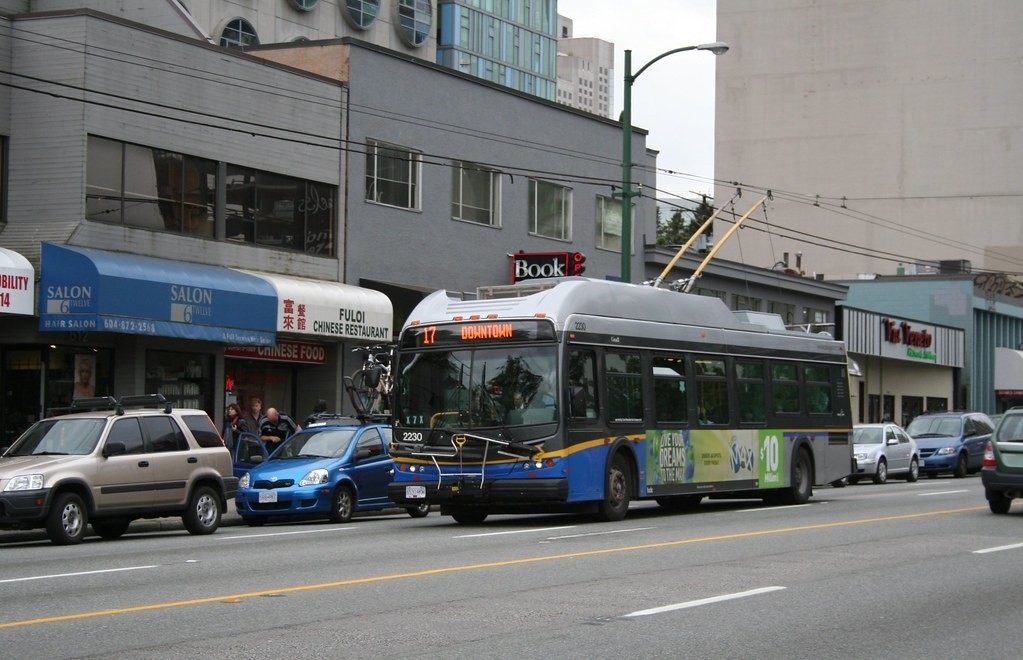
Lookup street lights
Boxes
[620,41,731,287]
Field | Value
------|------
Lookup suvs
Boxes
[0,393,241,547]
[980,407,1023,514]
[904,410,996,480]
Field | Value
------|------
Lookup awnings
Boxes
[39,240,279,347]
[0,248,34,316]
[848,356,863,377]
[994,346,1023,390]
[228,266,393,342]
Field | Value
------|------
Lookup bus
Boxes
[363,188,857,527]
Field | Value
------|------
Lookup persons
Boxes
[304,399,329,421]
[75,360,94,397]
[221,397,303,461]
[514,392,526,409]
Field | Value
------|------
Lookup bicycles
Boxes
[343,343,395,417]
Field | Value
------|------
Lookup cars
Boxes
[233,423,432,527]
[831,422,920,488]
